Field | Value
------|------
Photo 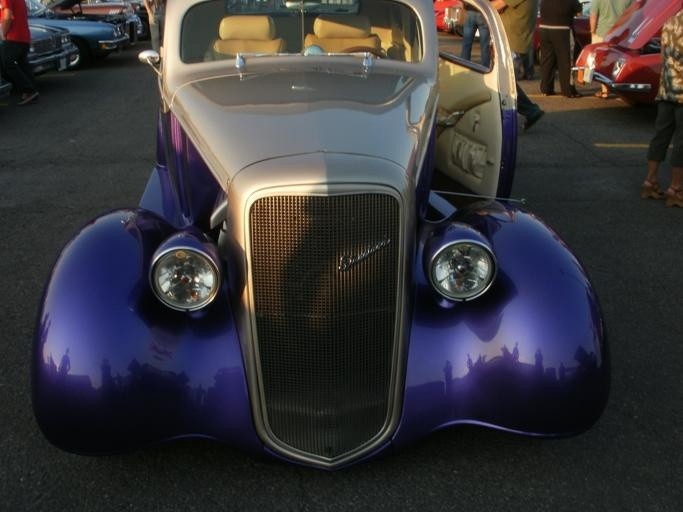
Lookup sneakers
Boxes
[525,109,544,129]
[18,90,40,105]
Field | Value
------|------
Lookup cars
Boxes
[569,1,682,123]
[442,6,480,42]
[26,21,79,80]
[0,77,12,107]
[23,1,129,71]
[488,1,592,71]
[37,1,141,50]
[30,1,610,473]
[433,1,464,35]
[129,1,165,41]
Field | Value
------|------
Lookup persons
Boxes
[589,1,632,99]
[492,0,544,129]
[0,0,39,106]
[640,9,683,207]
[459,1,489,68]
[539,1,583,97]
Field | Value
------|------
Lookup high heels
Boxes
[641,179,665,199]
[665,185,683,207]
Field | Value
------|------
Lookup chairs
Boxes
[304,14,381,57]
[214,14,285,57]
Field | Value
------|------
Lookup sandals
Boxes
[594,90,608,99]
